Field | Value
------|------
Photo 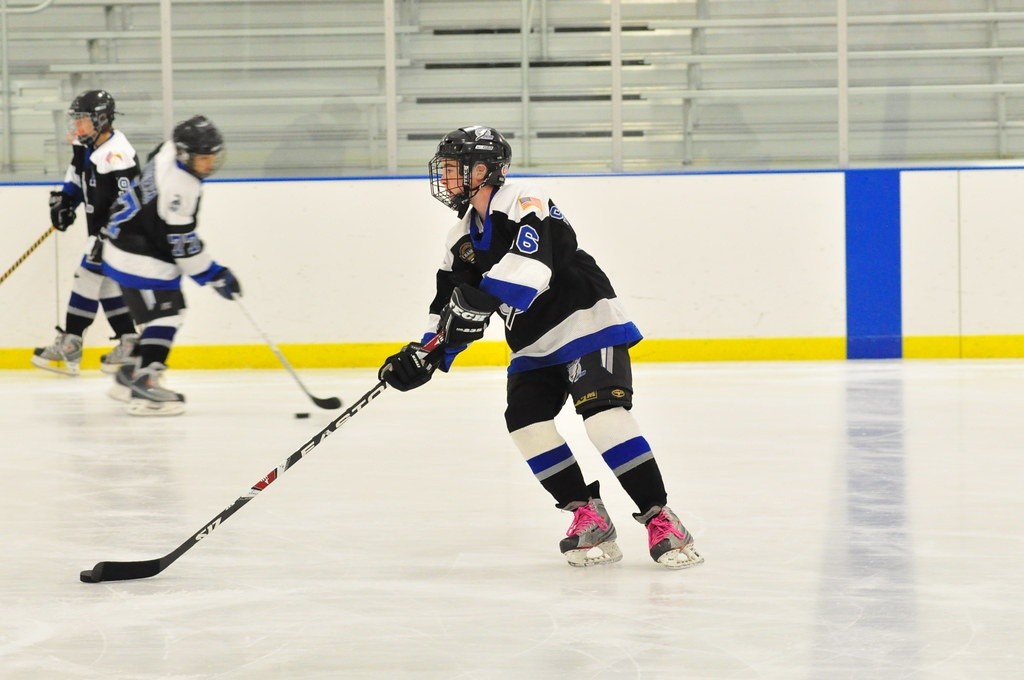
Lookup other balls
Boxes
[294,413,310,418]
[80,570,92,582]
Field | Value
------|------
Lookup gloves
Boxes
[437,287,492,347]
[378,342,442,392]
[208,267,246,301]
[48,191,76,231]
[87,232,106,263]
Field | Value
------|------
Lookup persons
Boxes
[31,91,141,375]
[380,125,703,571]
[101,114,244,417]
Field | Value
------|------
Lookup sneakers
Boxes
[32,326,84,375]
[100,334,141,374]
[632,503,705,570]
[108,364,134,402]
[555,480,623,567]
[127,361,187,416]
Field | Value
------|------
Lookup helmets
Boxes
[69,89,115,132]
[173,114,224,165]
[436,125,512,185]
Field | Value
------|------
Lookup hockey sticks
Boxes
[89,335,393,583]
[232,292,342,410]
[0,227,56,284]
